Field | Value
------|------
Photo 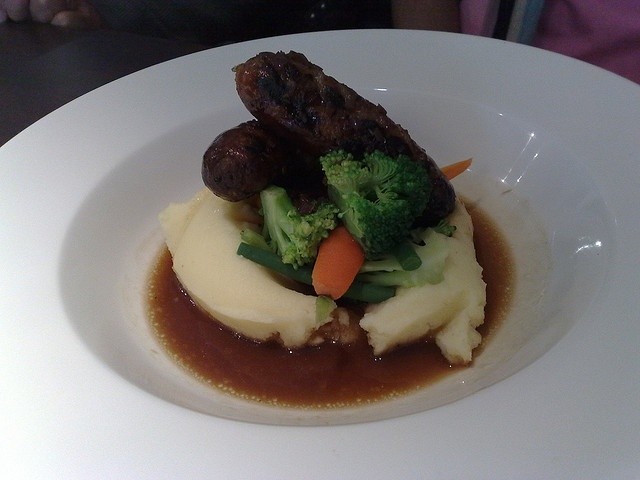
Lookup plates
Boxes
[0,28,639,477]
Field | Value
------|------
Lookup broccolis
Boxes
[259,186,339,271]
[320,148,432,261]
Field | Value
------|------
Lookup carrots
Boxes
[312,224,364,299]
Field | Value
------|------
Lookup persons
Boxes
[391,1,640,83]
[0,1,100,30]
